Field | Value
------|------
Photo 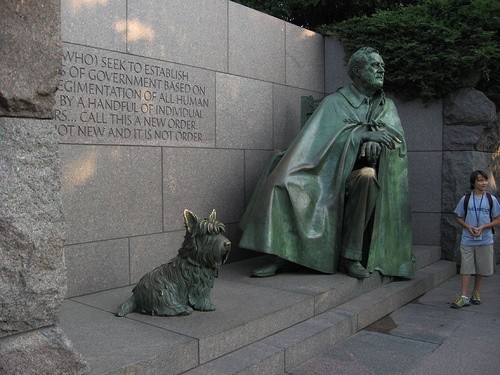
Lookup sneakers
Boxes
[451,295,471,308]
[472,289,481,304]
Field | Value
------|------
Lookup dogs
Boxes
[113,209,231,318]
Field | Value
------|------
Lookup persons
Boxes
[451,169,500,309]
[250,46,416,280]
